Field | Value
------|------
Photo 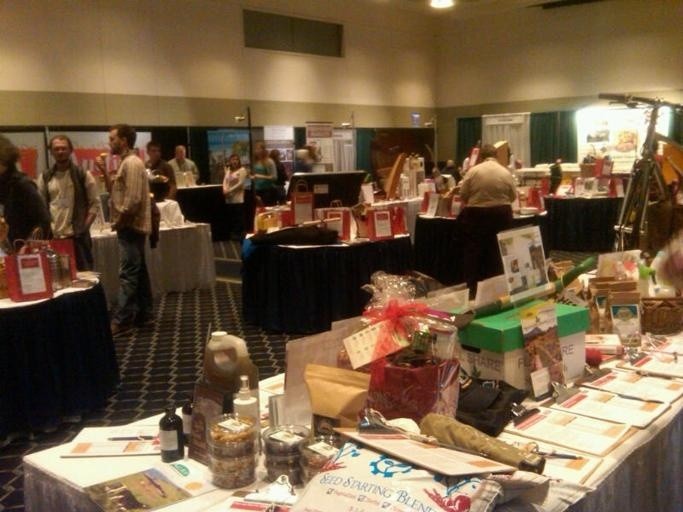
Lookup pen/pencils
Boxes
[535,451,583,461]
[107,435,156,441]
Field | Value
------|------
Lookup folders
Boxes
[574,364,683,404]
[540,380,671,430]
[641,331,683,355]
[503,402,638,457]
[616,345,683,378]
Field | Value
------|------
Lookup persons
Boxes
[222,154,248,246]
[34,134,101,273]
[143,142,177,201]
[167,144,199,181]
[249,141,278,207]
[0,133,54,252]
[460,144,517,279]
[107,126,156,339]
[148,175,185,228]
[270,149,289,206]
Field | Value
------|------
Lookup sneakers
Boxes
[135,312,153,324]
[112,320,132,337]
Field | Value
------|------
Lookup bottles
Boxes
[181,396,194,445]
[158,405,183,464]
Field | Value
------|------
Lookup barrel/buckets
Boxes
[204,331,259,414]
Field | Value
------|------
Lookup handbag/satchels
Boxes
[51,238,76,278]
[611,179,625,198]
[5,239,52,302]
[594,156,603,177]
[450,194,462,215]
[325,199,353,243]
[528,188,545,211]
[470,139,481,167]
[367,211,393,242]
[421,192,430,213]
[291,179,314,225]
[254,195,294,233]
[390,205,406,235]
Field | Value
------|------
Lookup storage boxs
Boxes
[457,301,592,394]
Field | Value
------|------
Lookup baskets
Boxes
[641,301,682,334]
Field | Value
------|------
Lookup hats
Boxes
[152,174,169,182]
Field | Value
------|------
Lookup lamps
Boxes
[234,106,250,122]
[425,116,436,127]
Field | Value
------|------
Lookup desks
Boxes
[100,185,226,241]
[23,333,683,512]
[242,224,411,335]
[543,195,626,251]
[415,213,549,279]
[0,272,121,430]
[87,223,213,312]
